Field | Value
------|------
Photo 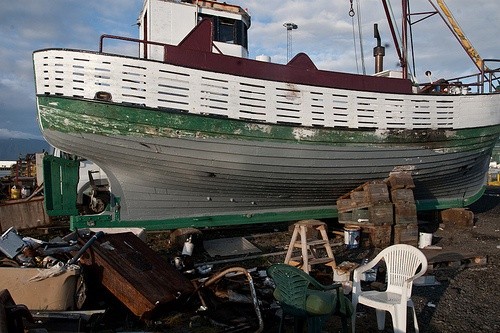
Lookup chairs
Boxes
[266,263,347,333]
[351,244,427,333]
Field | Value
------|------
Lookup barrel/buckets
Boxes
[419,232,432,249]
[343,226,362,249]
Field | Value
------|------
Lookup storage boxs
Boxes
[0,267,81,312]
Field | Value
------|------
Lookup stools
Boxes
[283,220,337,276]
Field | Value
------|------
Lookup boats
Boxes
[32,0,500,233]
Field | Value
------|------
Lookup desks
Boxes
[419,91,464,96]
[0,196,50,232]
